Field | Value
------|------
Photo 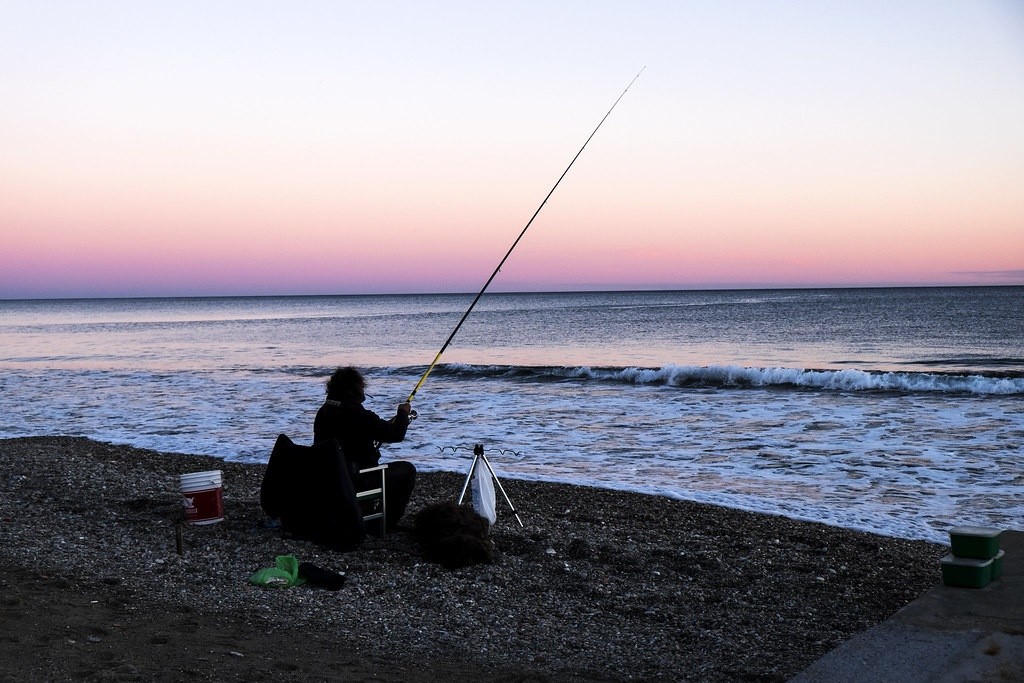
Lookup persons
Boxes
[314,365,417,531]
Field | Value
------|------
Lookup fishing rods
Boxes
[375,62,650,451]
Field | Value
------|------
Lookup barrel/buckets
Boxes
[179,470,224,525]
